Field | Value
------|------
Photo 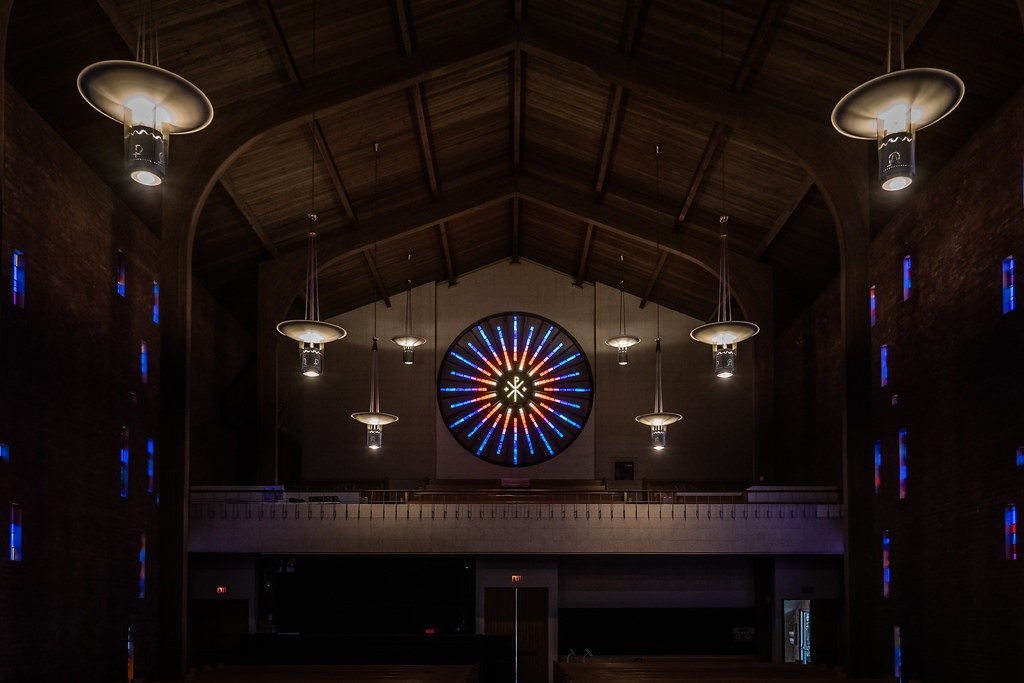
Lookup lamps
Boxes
[349,285,401,450]
[77,2,216,185]
[604,255,641,365]
[637,242,683,450]
[692,169,761,379]
[391,253,426,364]
[276,93,350,378]
[831,1,965,191]
